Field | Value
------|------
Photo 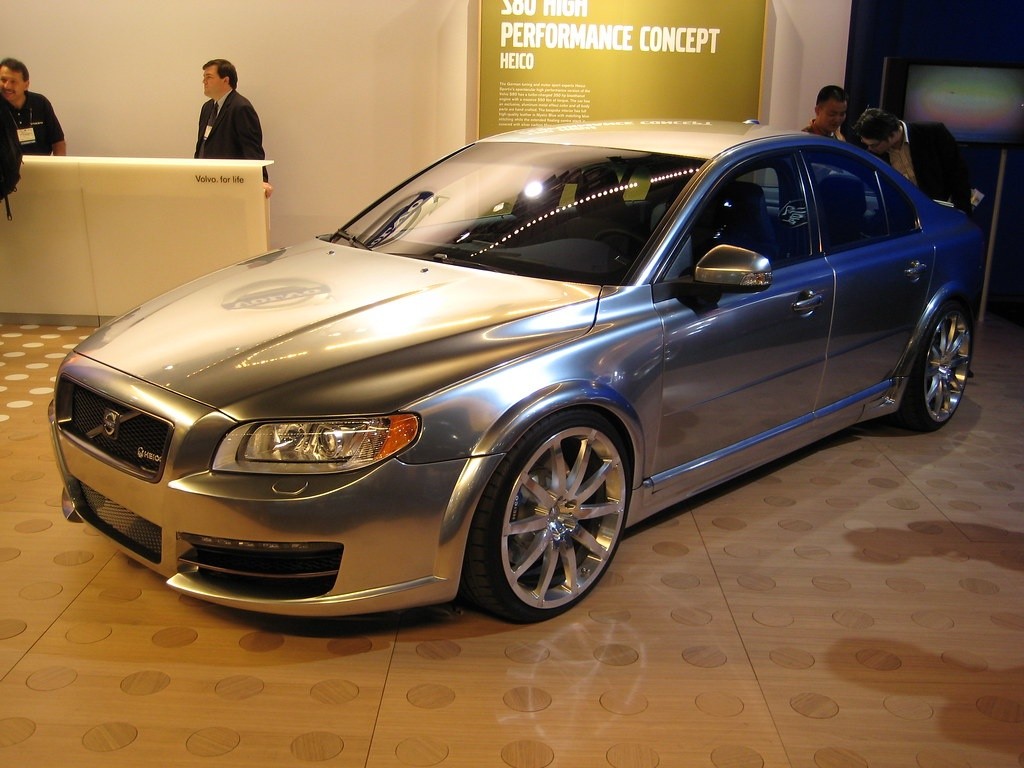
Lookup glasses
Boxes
[861,139,882,148]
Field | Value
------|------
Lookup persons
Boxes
[801,86,973,377]
[0,58,68,156]
[194,58,273,198]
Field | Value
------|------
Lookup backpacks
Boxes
[0,100,23,222]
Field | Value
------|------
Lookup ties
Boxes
[209,102,219,122]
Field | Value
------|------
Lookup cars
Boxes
[46,120,988,625]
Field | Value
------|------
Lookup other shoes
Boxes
[967,370,973,378]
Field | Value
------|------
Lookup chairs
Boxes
[558,166,629,250]
[703,182,773,251]
[818,173,872,245]
